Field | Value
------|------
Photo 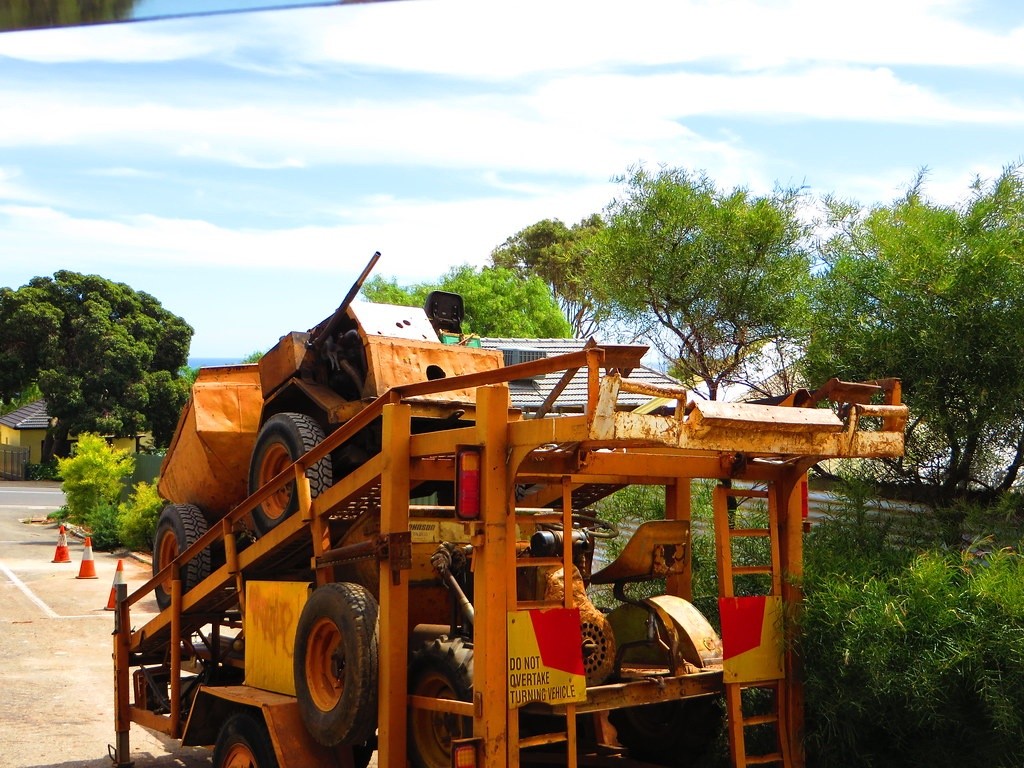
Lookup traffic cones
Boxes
[76,537,99,579]
[104,560,128,610]
[51,524,72,564]
